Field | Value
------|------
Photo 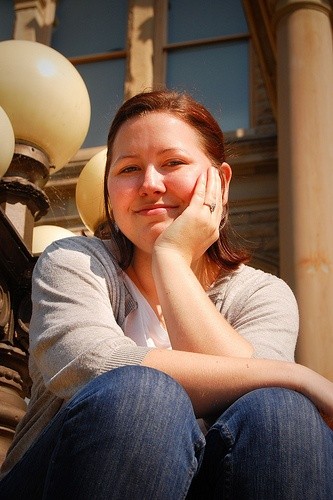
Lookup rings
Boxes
[203,200,216,212]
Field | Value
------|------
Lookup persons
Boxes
[0,90,333,500]
